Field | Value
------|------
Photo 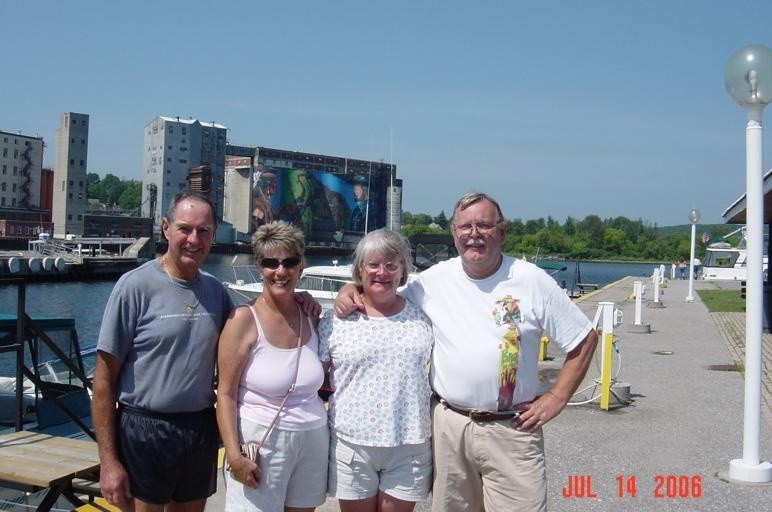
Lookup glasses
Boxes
[454,222,502,230]
[362,262,399,273]
[261,257,301,269]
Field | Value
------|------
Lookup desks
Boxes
[0,431,101,512]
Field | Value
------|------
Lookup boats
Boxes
[699,225,768,283]
[222,254,435,310]
[0,311,99,451]
[533,259,579,302]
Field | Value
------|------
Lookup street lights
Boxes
[724,44,771,483]
[686,209,700,302]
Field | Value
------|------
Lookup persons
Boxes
[677,256,687,280]
[351,175,375,231]
[694,256,701,281]
[252,169,304,234]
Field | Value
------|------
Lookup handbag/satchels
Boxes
[226,443,260,471]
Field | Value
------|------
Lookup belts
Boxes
[431,388,525,422]
